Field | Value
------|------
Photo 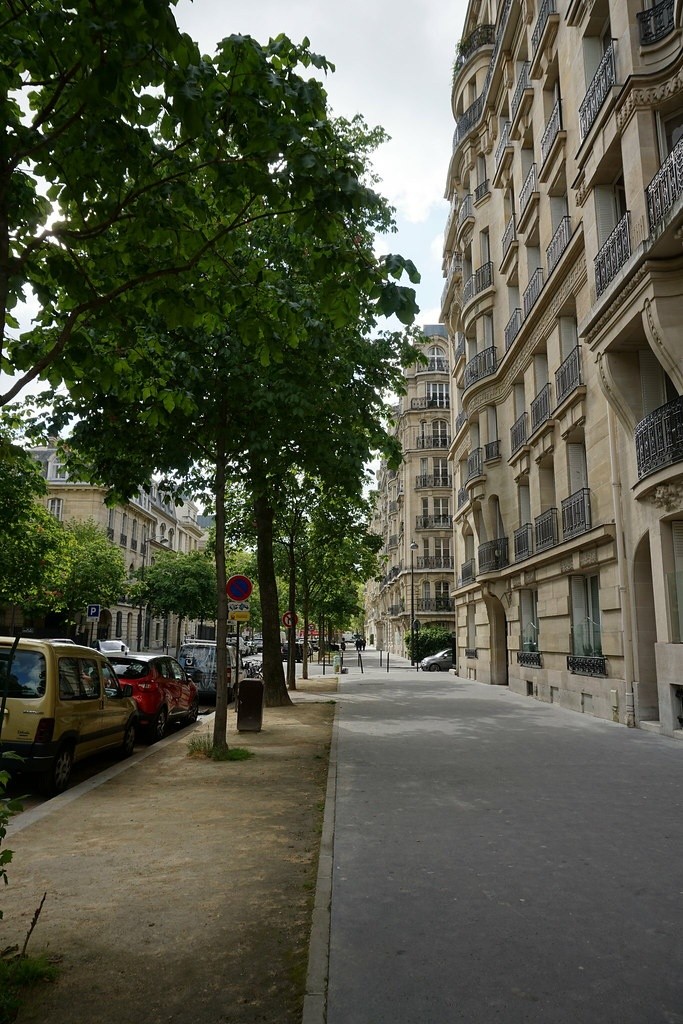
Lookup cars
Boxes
[280,639,319,662]
[0,636,139,793]
[177,643,249,704]
[421,648,456,672]
[226,636,263,656]
[90,639,130,653]
[82,652,200,740]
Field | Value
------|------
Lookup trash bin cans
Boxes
[237,677,263,731]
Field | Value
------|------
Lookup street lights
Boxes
[137,535,169,651]
[409,537,419,666]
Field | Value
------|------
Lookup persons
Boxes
[356,637,366,651]
[341,638,346,651]
